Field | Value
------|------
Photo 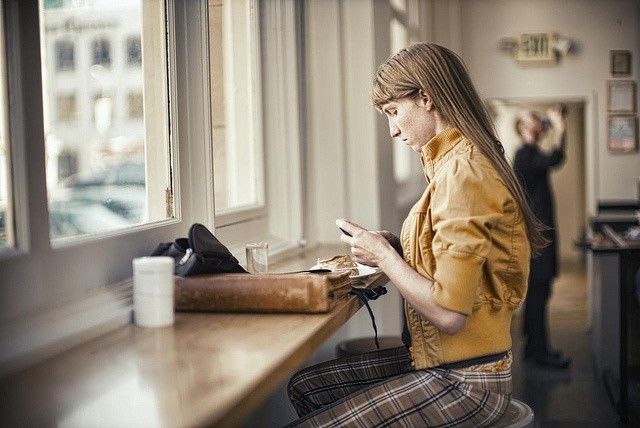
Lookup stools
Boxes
[336,335,404,357]
[484,399,535,428]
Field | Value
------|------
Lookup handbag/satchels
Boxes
[150,222,248,276]
[173,268,354,314]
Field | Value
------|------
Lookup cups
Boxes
[128,255,175,328]
[246,243,268,273]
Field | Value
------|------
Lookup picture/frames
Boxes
[606,79,637,113]
[607,112,637,153]
[611,51,632,76]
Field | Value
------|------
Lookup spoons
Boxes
[266,268,333,275]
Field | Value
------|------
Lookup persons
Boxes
[277,42,553,428]
[512,104,573,375]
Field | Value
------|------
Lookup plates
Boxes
[311,265,384,285]
